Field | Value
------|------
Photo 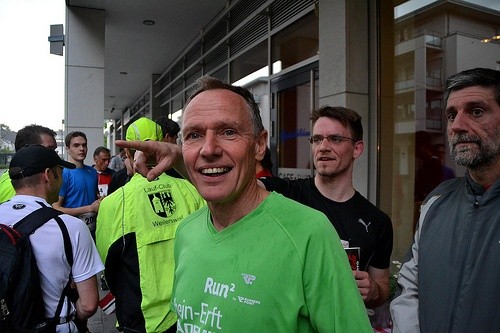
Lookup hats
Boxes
[9,144,76,180]
[126,117,163,150]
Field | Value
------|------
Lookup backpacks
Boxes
[0,207,65,333]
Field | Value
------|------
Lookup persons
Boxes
[390,68,500,333]
[0,124,118,333]
[92,75,394,333]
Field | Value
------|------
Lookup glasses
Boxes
[308,135,360,144]
